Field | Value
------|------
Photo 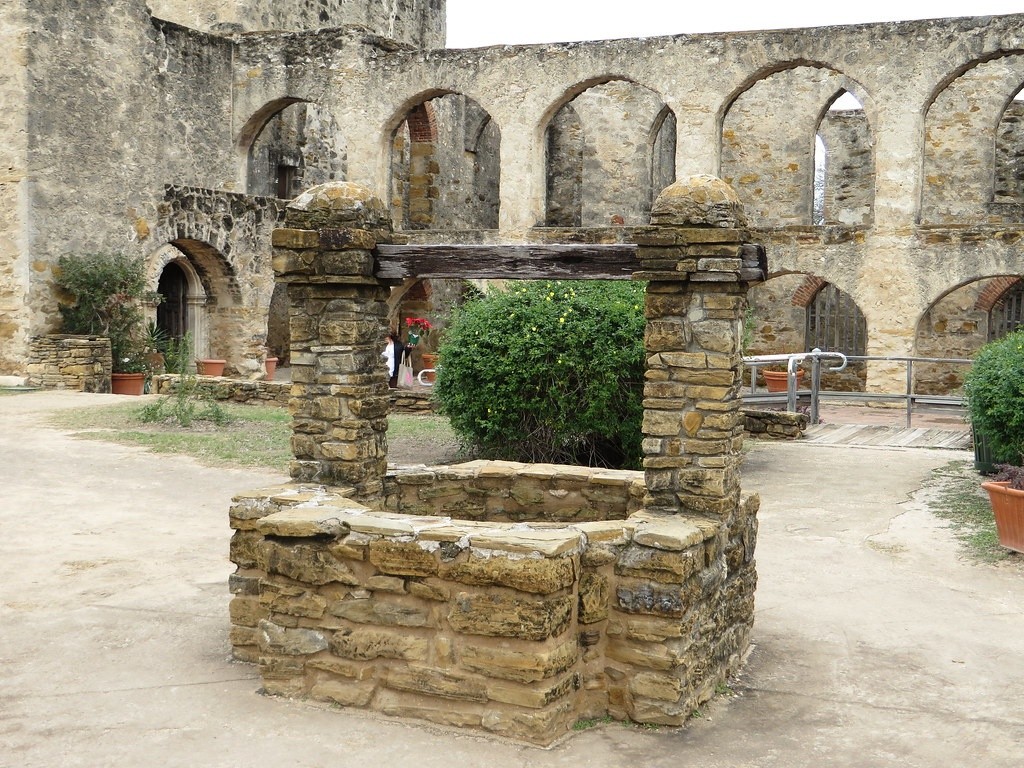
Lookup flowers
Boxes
[406,317,431,337]
[115,351,148,374]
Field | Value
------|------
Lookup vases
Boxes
[111,373,145,395]
[422,352,440,380]
[264,357,279,379]
[197,359,226,376]
[408,333,419,345]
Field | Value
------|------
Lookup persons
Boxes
[381,324,417,388]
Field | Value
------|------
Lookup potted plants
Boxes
[957,326,1024,557]
[762,362,804,393]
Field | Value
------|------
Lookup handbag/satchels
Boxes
[397,350,413,391]
